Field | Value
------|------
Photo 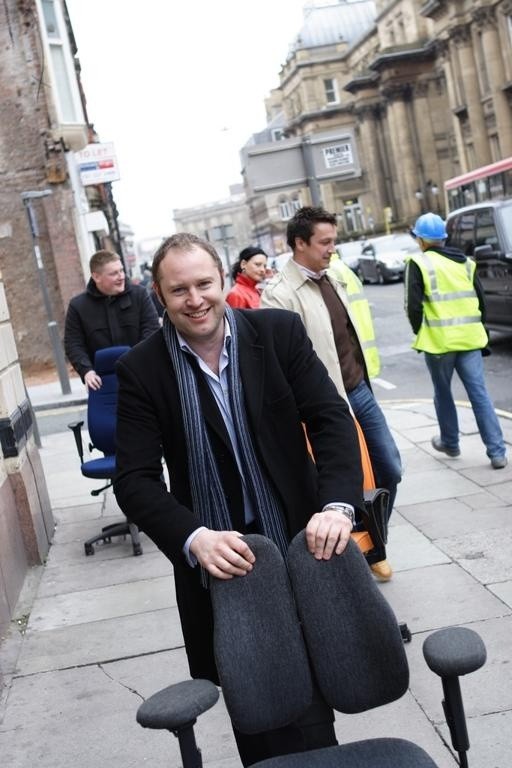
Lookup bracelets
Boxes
[322,504,357,520]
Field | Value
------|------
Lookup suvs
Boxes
[443,196,511,334]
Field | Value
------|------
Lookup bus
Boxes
[446,155,512,220]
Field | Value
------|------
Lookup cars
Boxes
[262,229,424,285]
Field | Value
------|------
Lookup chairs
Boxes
[299,406,388,566]
[134,527,488,767]
[67,345,144,556]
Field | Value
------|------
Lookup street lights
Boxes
[414,188,427,216]
[431,183,442,216]
[22,187,74,397]
[337,207,376,234]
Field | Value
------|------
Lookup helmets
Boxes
[413,212,448,240]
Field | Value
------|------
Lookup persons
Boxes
[109,231,368,767]
[404,211,508,468]
[223,245,268,310]
[257,203,404,584]
[63,246,163,395]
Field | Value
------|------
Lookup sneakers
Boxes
[432,434,459,456]
[370,558,391,580]
[490,454,508,469]
